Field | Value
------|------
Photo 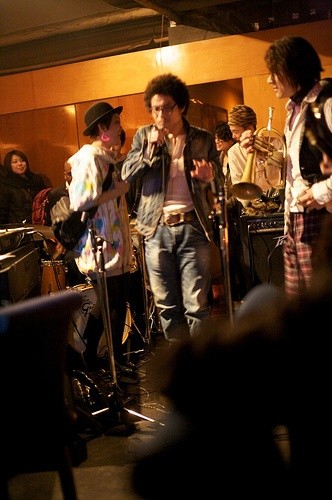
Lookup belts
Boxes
[158,211,197,226]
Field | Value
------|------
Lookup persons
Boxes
[44,160,85,287]
[68,101,132,369]
[121,75,226,349]
[0,150,52,226]
[215,123,237,193]
[264,36,332,298]
[110,128,127,173]
[228,104,283,207]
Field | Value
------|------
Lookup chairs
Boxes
[0,291,85,500]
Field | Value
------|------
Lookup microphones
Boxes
[149,126,165,162]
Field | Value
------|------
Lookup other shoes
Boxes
[117,357,136,370]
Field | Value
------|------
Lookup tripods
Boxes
[70,205,165,428]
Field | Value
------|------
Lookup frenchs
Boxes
[227,106,287,200]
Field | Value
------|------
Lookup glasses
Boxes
[150,103,176,113]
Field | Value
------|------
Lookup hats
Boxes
[227,105,257,129]
[83,101,123,137]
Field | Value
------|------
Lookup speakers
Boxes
[240,213,285,292]
